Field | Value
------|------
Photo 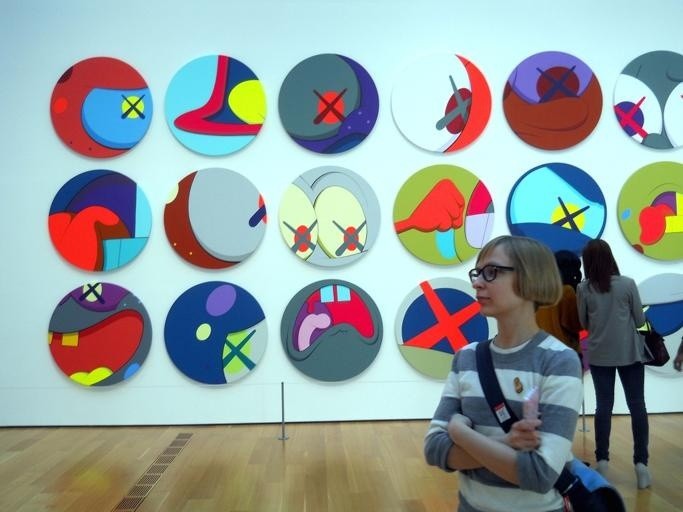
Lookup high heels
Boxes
[587,458,609,477]
[633,462,652,489]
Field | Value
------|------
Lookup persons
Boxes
[534,248,582,355]
[673,336,683,374]
[575,237,654,491]
[422,234,586,511]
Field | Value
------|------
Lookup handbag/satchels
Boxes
[636,323,670,366]
[554,455,626,512]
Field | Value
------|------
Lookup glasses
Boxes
[468,264,516,283]
